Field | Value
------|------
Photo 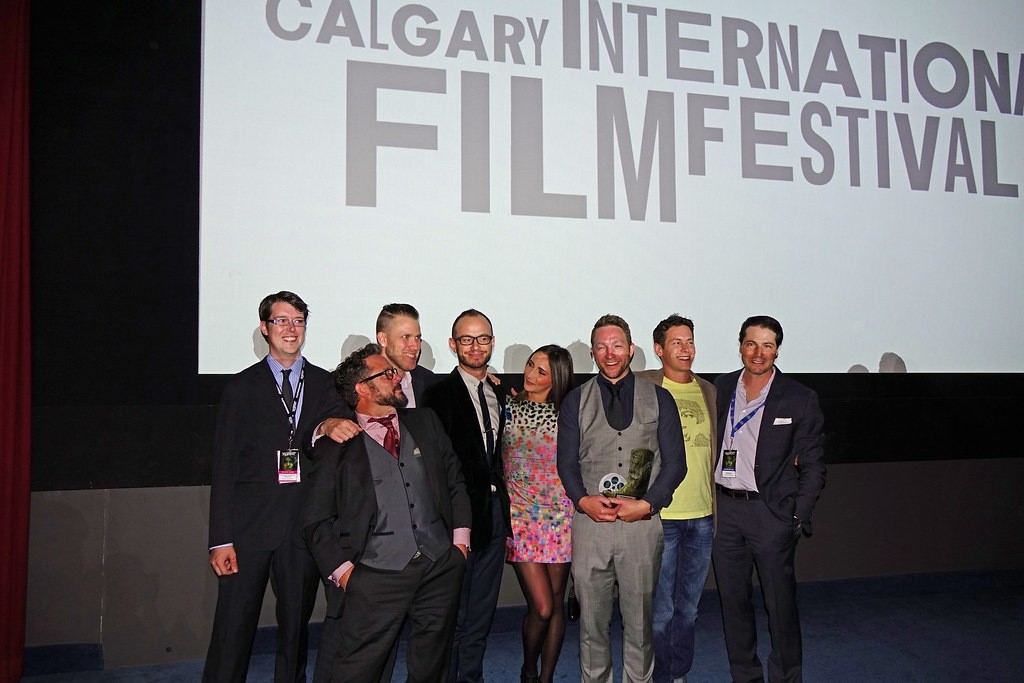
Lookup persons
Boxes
[497,344,572,683]
[299,342,472,683]
[557,314,688,683]
[281,457,297,471]
[714,316,828,683]
[303,303,501,462]
[203,292,331,683]
[726,456,735,469]
[631,315,718,683]
[426,308,514,683]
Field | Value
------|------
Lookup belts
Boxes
[716,483,760,501]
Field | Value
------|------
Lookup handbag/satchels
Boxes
[568,567,580,622]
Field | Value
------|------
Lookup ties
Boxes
[478,381,494,471]
[603,382,624,430]
[367,414,400,460]
[281,369,293,412]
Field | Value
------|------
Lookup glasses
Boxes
[455,335,491,345]
[354,368,398,395]
[266,317,307,327]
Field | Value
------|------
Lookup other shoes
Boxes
[521,663,538,683]
